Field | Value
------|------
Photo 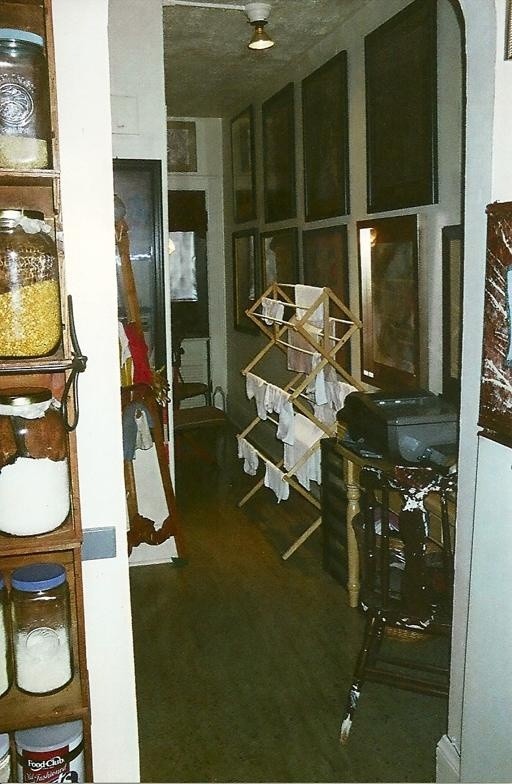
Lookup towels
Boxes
[283,413,323,491]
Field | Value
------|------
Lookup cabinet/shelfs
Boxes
[0,0,96,784]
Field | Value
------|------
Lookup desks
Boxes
[321,439,455,624]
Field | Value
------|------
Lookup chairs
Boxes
[337,467,458,747]
[172,405,232,471]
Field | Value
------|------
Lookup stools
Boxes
[352,509,439,610]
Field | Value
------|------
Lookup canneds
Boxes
[0,733,11,783]
[12,720,86,783]
